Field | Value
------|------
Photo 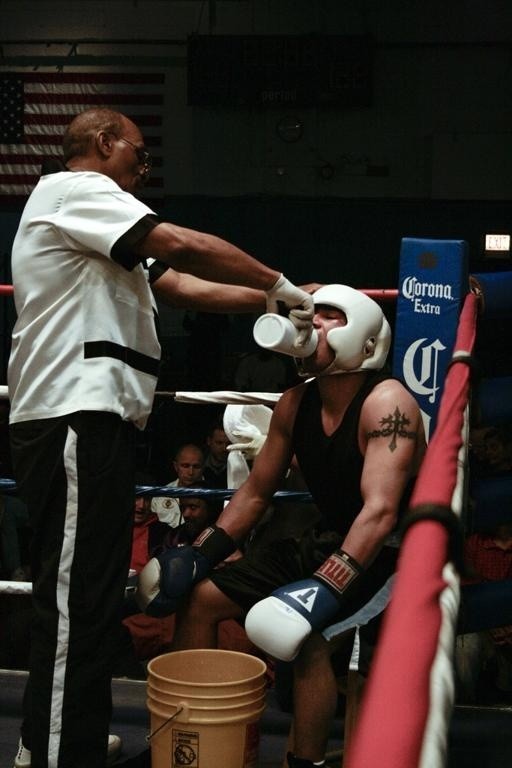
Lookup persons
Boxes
[8,105,327,766]
[139,275,432,766]
[127,404,512,718]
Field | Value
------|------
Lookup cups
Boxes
[251,311,319,361]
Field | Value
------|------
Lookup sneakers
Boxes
[13,735,123,768]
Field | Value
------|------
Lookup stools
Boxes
[280,645,378,768]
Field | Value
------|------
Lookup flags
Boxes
[0,70,175,214]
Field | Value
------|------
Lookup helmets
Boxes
[293,283,392,377]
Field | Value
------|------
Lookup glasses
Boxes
[110,129,152,185]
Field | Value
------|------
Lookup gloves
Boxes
[244,548,366,663]
[226,430,268,461]
[264,273,315,350]
[137,524,238,618]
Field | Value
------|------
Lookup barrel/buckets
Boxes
[145,648,267,768]
